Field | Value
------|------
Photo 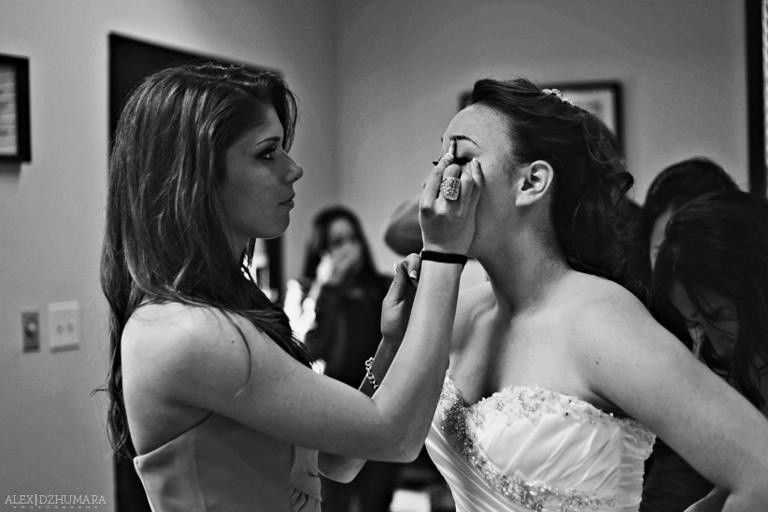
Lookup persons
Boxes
[394,78,768,512]
[650,192,767,512]
[287,206,395,390]
[92,62,487,510]
[630,156,754,310]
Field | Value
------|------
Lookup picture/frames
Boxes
[460,82,622,164]
[0,53,29,162]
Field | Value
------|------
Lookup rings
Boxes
[441,177,462,201]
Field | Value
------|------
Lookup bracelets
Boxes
[366,356,378,394]
[420,248,468,266]
